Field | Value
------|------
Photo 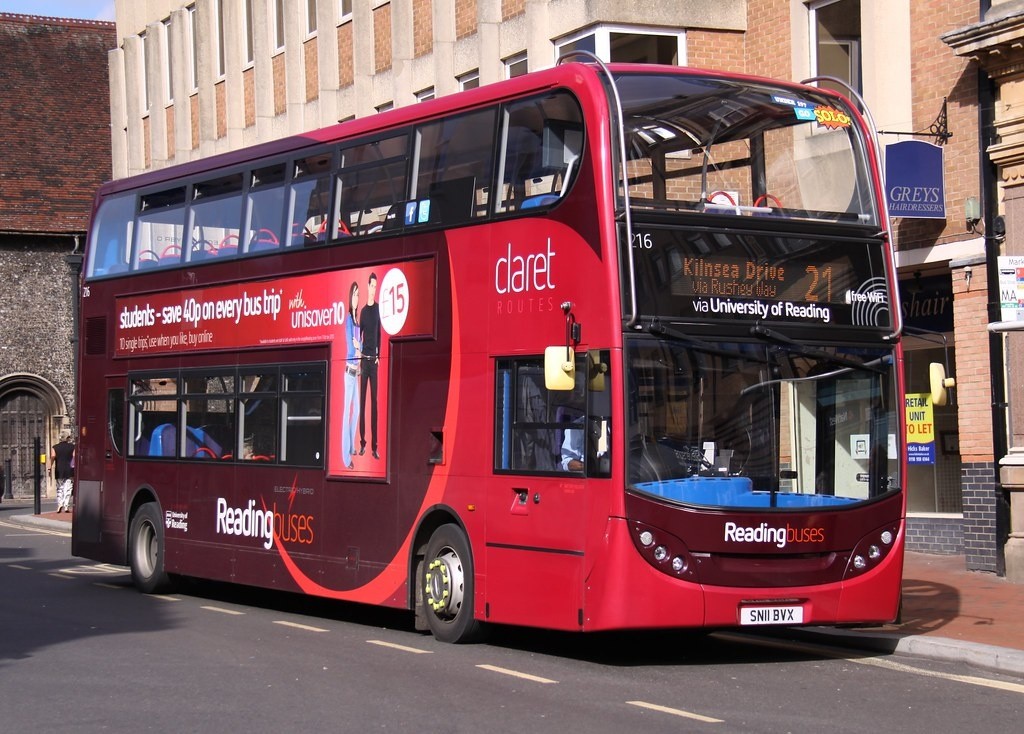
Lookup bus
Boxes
[70,49,955,646]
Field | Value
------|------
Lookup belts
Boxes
[345,366,358,377]
[363,355,377,361]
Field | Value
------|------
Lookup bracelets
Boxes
[48,469,51,470]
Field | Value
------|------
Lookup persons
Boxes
[560,393,611,471]
[48,430,77,513]
[341,274,381,470]
[0,461,5,504]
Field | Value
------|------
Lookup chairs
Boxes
[138,250,159,270]
[316,218,351,241]
[148,423,229,458]
[752,194,789,220]
[217,234,240,257]
[554,406,585,472]
[290,224,317,246]
[156,245,182,266]
[249,229,280,253]
[191,240,218,262]
[702,192,743,216]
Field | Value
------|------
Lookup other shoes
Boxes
[57,504,70,513]
[348,462,355,470]
[353,450,358,456]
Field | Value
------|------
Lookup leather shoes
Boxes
[358,448,366,455]
[372,450,381,461]
